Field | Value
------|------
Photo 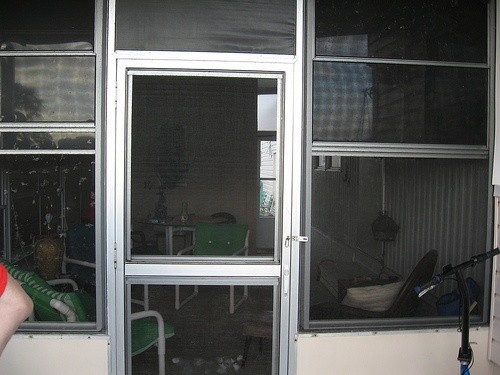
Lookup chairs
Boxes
[60,226,149,311]
[240,304,273,368]
[0,257,165,375]
[310,250,438,320]
[176,223,250,314]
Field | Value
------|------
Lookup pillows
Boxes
[342,281,404,313]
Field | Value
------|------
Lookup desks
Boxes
[134,216,232,255]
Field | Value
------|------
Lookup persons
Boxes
[0,263,34,356]
[32,234,76,293]
